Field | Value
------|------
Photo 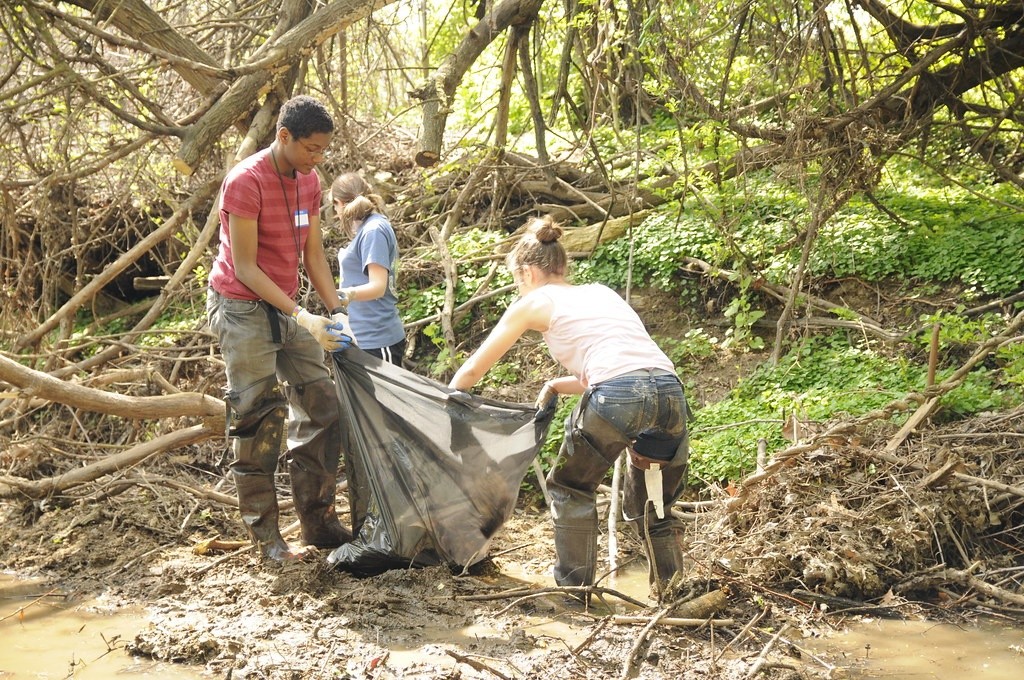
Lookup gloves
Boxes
[290,305,359,352]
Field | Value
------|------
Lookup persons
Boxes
[204,92,361,566]
[329,173,407,368]
[448,212,690,608]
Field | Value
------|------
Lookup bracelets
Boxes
[291,305,304,320]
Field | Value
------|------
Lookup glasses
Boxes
[297,138,332,156]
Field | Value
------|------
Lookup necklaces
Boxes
[265,147,305,308]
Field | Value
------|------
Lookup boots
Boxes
[223,374,354,570]
[546,422,612,603]
[627,431,687,596]
